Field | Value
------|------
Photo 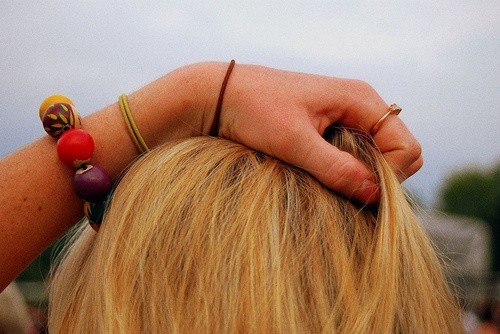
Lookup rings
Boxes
[369,103,402,138]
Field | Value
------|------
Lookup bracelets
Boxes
[39,94,114,234]
[118,92,150,155]
[209,59,236,137]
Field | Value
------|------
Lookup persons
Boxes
[0,57,467,334]
